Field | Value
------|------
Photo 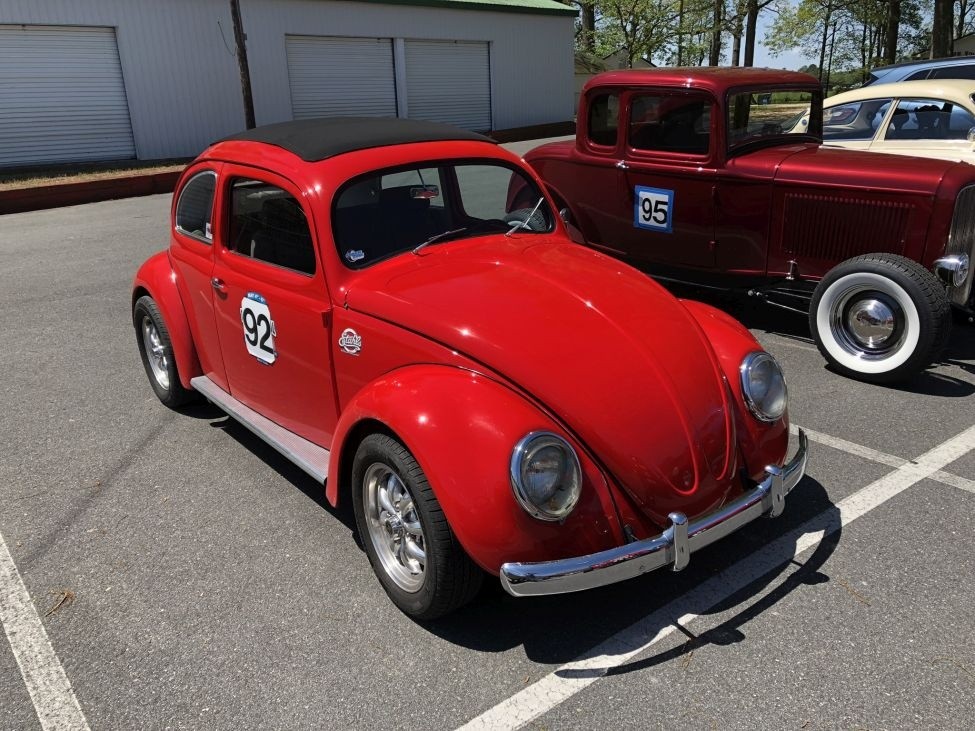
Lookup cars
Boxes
[505,67,975,386]
[776,55,975,166]
[130,116,810,621]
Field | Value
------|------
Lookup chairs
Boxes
[916,105,941,137]
[250,195,316,272]
[377,195,431,259]
[884,108,911,138]
